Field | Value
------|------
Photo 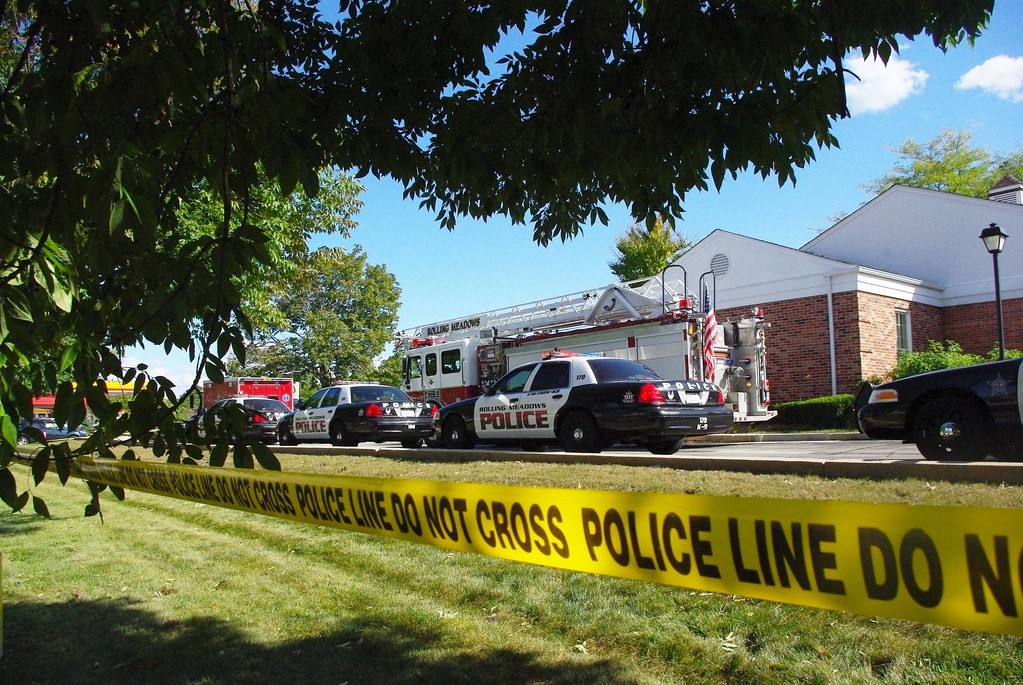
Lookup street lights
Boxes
[979,222,1009,363]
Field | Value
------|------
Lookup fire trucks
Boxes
[190,377,300,413]
[400,253,778,422]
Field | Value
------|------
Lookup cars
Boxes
[184,394,293,444]
[276,381,435,448]
[852,357,1023,462]
[430,348,733,455]
[17,418,88,445]
